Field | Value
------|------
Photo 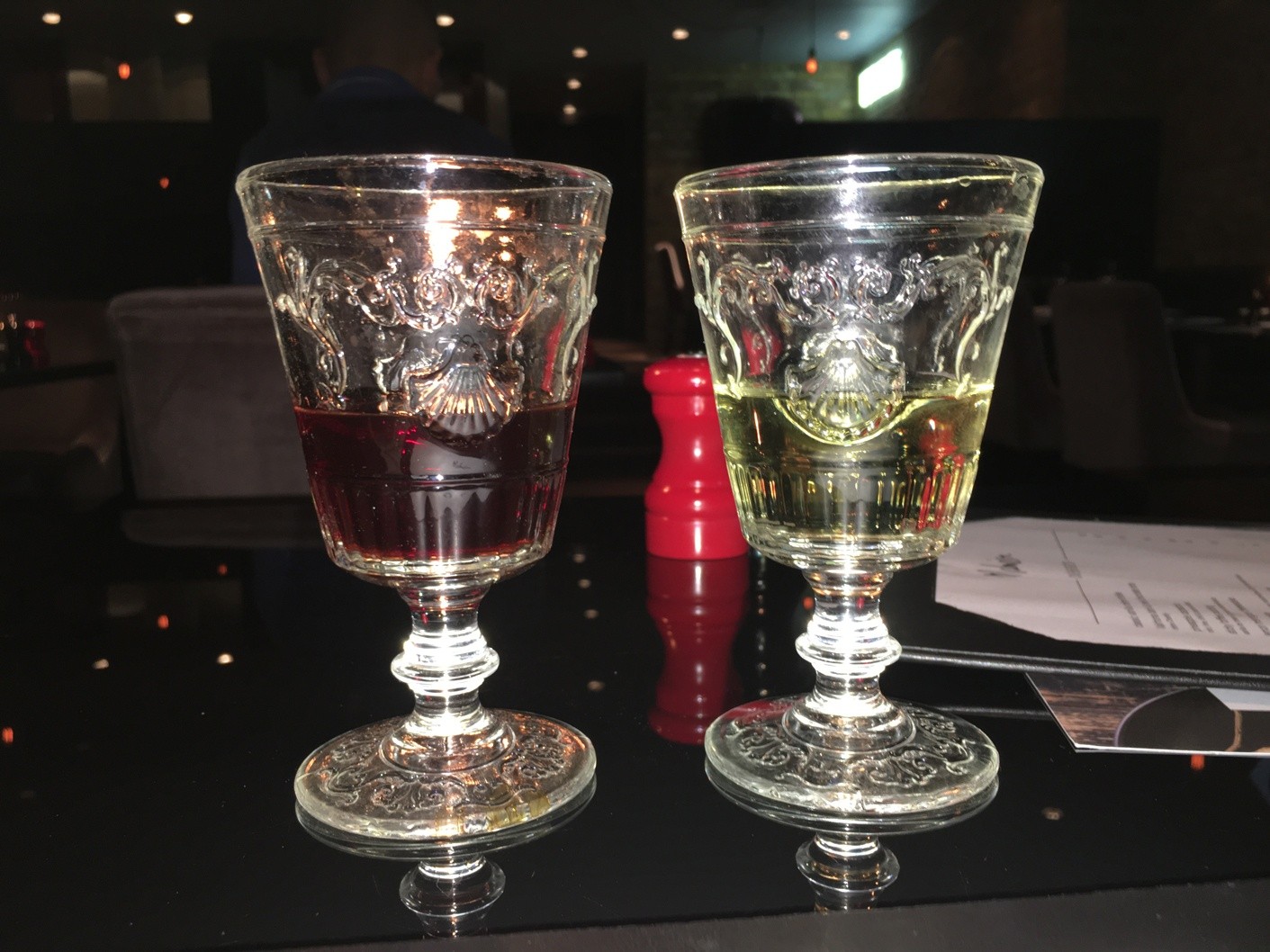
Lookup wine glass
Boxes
[673,152,1044,814]
[235,152,613,840]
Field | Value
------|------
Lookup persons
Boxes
[665,97,800,351]
[225,0,512,285]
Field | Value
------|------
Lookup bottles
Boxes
[643,358,749,559]
[646,559,750,745]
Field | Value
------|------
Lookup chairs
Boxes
[106,287,315,546]
